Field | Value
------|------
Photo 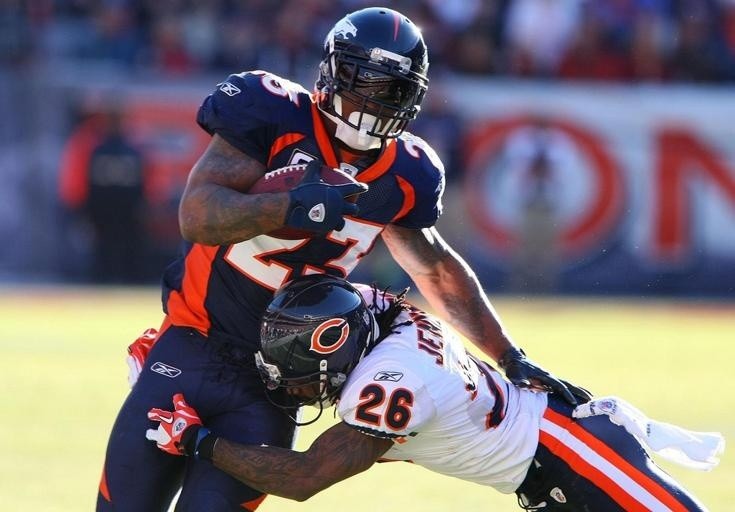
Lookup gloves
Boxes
[500,348,593,409]
[127,328,158,389]
[146,395,220,464]
[285,160,369,233]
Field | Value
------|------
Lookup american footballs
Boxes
[249,162,360,240]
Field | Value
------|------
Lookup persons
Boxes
[123,272,730,510]
[86,4,596,512]
[0,0,735,299]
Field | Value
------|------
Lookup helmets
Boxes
[315,7,430,112]
[257,275,381,408]
